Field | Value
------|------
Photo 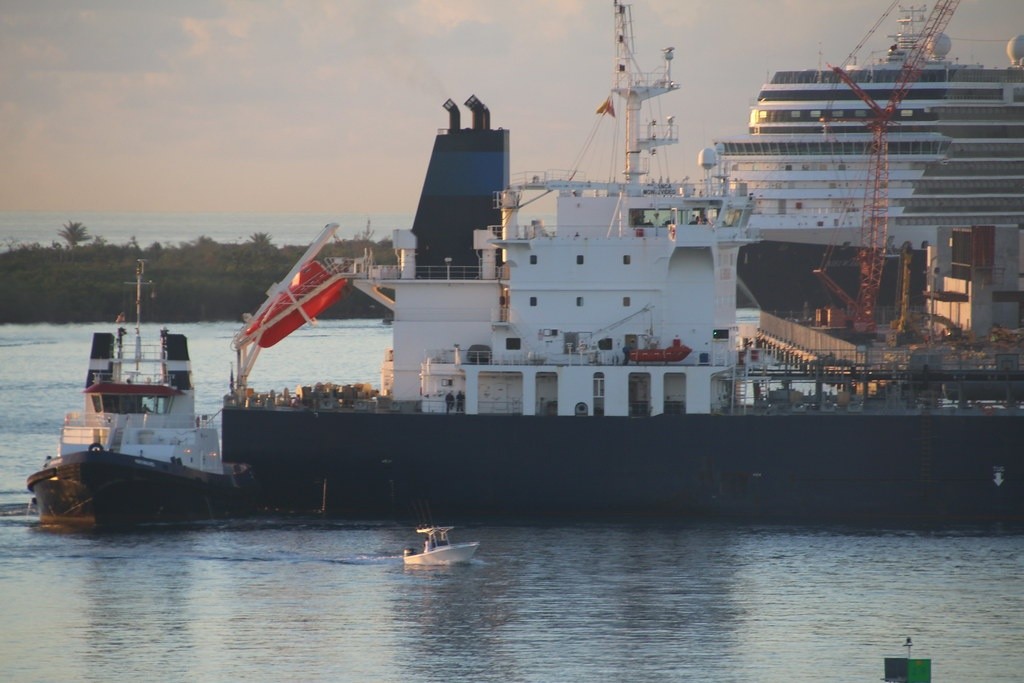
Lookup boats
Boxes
[404,515,483,569]
[26,255,224,534]
[626,338,690,363]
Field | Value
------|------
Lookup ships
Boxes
[222,0,1024,534]
